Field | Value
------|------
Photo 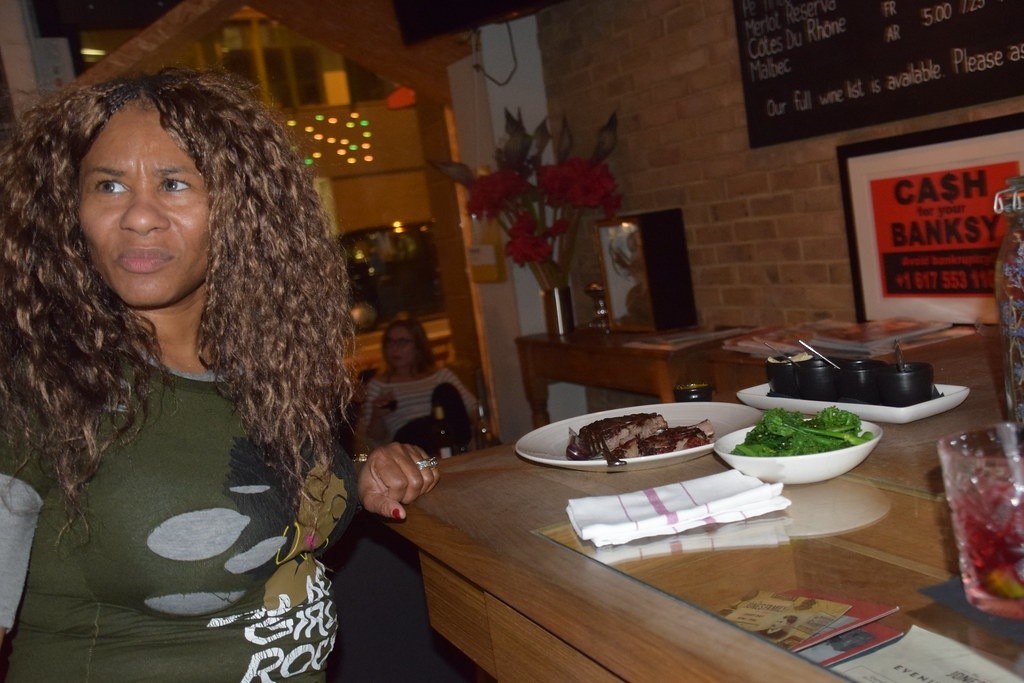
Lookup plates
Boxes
[513,402,763,472]
[737,366,971,424]
[714,415,885,485]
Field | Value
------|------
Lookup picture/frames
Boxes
[835,112,1023,324]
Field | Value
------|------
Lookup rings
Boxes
[416,459,431,470]
[428,456,438,468]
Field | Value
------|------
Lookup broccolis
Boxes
[731,405,871,458]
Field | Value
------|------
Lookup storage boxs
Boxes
[591,206,699,336]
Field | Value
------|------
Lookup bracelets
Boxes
[351,453,368,462]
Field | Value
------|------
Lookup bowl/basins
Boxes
[763,340,938,406]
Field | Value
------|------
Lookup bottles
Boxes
[430,400,454,459]
[471,395,489,449]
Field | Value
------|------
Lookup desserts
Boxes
[577,413,715,461]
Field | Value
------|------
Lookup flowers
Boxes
[423,105,624,285]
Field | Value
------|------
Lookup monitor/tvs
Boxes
[392,0,568,47]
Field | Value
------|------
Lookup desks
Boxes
[353,322,1024,683]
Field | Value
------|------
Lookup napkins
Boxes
[564,469,792,551]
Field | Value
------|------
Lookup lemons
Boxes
[980,566,1024,600]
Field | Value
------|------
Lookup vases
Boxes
[540,287,576,336]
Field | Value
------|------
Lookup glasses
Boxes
[384,338,415,349]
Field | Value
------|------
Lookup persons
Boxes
[358,319,479,458]
[0,67,440,683]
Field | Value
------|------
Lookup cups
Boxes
[938,422,1024,620]
